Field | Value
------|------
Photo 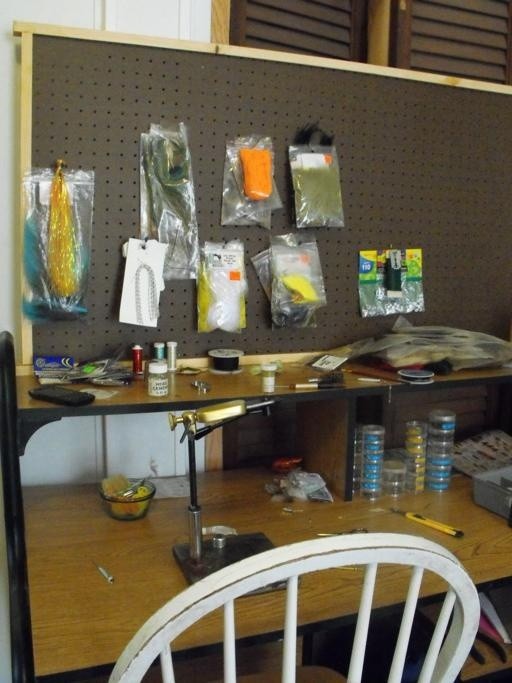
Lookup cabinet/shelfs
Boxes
[0,331,512,683]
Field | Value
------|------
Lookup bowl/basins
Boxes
[97,477,157,521]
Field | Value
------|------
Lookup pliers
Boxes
[435,596,507,663]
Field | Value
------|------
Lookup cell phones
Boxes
[28,386,97,406]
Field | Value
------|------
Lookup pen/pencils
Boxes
[290,383,346,390]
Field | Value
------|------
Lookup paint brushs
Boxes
[342,368,411,386]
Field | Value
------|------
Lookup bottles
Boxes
[146,360,168,398]
[261,363,276,394]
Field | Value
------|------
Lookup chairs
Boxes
[108,532,482,683]
